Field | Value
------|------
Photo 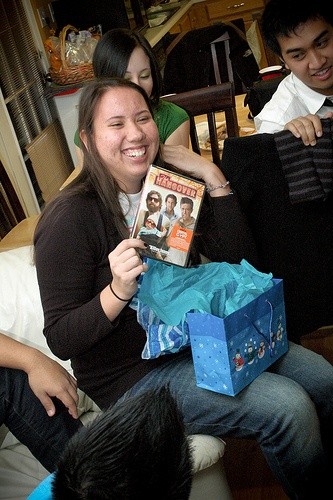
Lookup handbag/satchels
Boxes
[186,279,289,397]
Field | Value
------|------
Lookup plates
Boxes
[199,127,256,152]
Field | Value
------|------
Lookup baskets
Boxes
[48,24,102,86]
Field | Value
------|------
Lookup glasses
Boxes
[148,196,159,203]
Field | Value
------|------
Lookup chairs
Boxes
[219,117,333,341]
[162,81,238,168]
[159,21,264,162]
[244,76,286,117]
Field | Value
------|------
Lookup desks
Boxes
[53,0,283,171]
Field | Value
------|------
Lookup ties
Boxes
[323,98,333,107]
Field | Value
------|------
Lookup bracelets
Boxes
[206,181,231,195]
[109,283,131,302]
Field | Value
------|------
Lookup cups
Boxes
[258,65,284,80]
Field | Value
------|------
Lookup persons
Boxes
[27,382,198,500]
[0,333,86,474]
[57,29,190,193]
[33,79,333,500]
[253,0,333,146]
[138,190,196,260]
